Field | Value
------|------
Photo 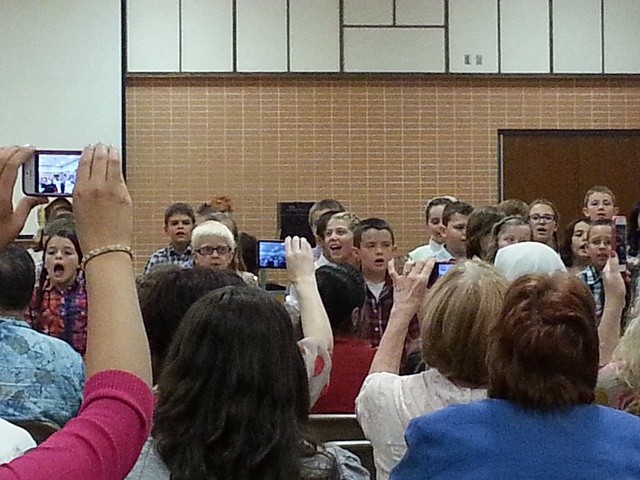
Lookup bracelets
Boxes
[81,244,135,270]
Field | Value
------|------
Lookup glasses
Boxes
[590,241,610,246]
[194,245,229,255]
[528,215,556,224]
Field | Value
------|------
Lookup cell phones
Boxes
[427,262,455,289]
[255,240,286,269]
[611,215,628,273]
[21,149,81,197]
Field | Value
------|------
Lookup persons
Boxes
[323,211,363,275]
[526,199,561,261]
[0,243,86,429]
[407,196,458,260]
[125,286,372,478]
[595,250,639,416]
[423,201,475,292]
[142,236,336,414]
[464,204,507,261]
[145,202,198,274]
[2,141,155,480]
[389,268,639,480]
[192,219,260,291]
[308,199,344,262]
[28,218,77,288]
[144,266,248,388]
[28,227,87,356]
[581,185,620,221]
[577,220,640,336]
[194,210,248,282]
[352,259,512,480]
[561,216,591,275]
[283,210,346,341]
[350,218,422,376]
[292,261,378,413]
[487,215,533,263]
